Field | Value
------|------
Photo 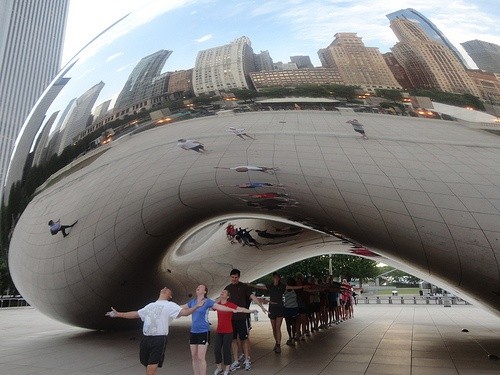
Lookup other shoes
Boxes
[274,345,277,349]
[276,348,280,352]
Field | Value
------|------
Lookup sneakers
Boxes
[214,369,223,375]
[224,370,230,375]
[243,359,252,370]
[230,361,241,371]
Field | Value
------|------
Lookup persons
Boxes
[212,166,275,174]
[295,268,365,342]
[283,275,314,346]
[105,287,208,375]
[219,182,284,188]
[209,289,259,375]
[179,284,242,375]
[224,127,254,140]
[176,138,208,153]
[223,193,381,257]
[246,272,311,354]
[48,219,79,237]
[224,269,271,372]
[237,299,268,365]
[346,118,367,139]
